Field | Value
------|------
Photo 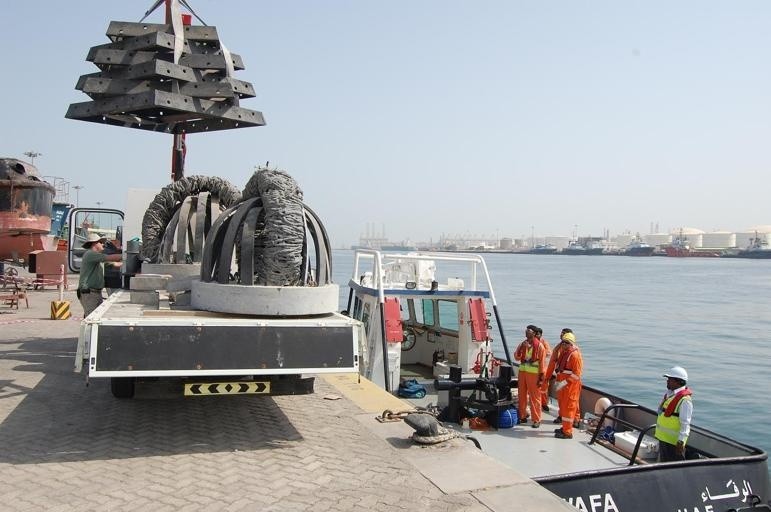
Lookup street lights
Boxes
[73,184,83,207]
[96,201,104,227]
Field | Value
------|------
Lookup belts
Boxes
[80,287,101,294]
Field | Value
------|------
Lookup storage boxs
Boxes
[614,430,660,458]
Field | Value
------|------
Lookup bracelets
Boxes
[677,440,683,445]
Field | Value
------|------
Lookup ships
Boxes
[352,225,418,251]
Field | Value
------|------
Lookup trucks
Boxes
[65,179,371,401]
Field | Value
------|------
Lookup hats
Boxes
[81,233,107,249]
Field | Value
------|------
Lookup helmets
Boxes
[563,332,575,343]
[663,366,688,381]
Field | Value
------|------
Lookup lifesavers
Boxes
[595,394,617,427]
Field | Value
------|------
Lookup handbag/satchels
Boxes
[81,288,90,293]
[77,289,80,299]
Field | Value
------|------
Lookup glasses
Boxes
[97,241,102,244]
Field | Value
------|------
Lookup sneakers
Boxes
[517,405,579,439]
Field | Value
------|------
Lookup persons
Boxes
[76,232,122,319]
[512,325,547,428]
[653,366,693,462]
[533,328,551,412]
[541,328,583,428]
[550,332,583,438]
[102,234,123,297]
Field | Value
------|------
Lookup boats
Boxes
[530,242,555,253]
[624,237,654,256]
[735,239,771,258]
[561,241,588,255]
[346,248,771,511]
[1,157,74,268]
[665,241,695,256]
